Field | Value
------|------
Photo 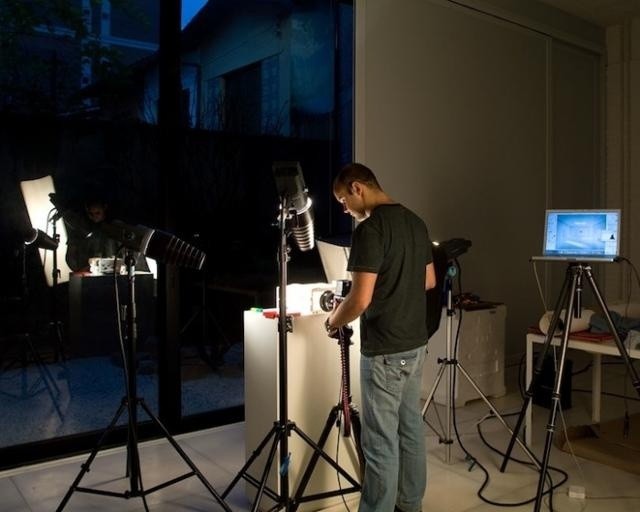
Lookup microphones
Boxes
[109,220,206,271]
[272,161,317,253]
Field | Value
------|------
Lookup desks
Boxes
[521,330,640,446]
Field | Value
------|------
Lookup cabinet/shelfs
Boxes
[419,301,509,409]
[243,307,366,511]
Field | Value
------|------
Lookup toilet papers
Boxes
[539,306,595,336]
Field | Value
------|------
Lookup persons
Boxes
[325,163,436,512]
[66,196,126,272]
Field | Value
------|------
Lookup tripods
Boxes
[0,215,81,423]
[219,256,365,512]
[419,282,545,475]
[51,279,233,512]
[487,266,640,512]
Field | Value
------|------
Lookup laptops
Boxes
[529,208,622,263]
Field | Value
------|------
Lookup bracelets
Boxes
[326,319,336,331]
